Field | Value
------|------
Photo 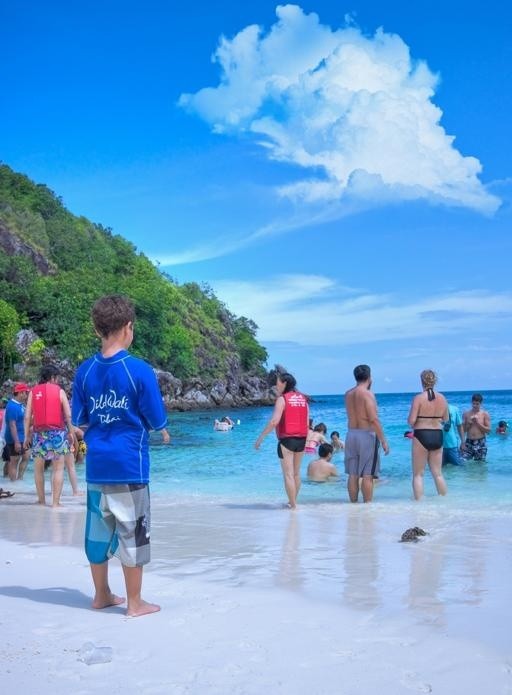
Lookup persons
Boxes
[496,420,507,434]
[69,293,170,618]
[254,371,310,510]
[408,368,449,501]
[459,393,491,463]
[439,404,466,468]
[222,417,235,426]
[303,365,390,503]
[0,365,87,509]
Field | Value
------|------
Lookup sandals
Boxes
[0,488,16,498]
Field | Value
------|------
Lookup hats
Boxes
[14,384,31,393]
